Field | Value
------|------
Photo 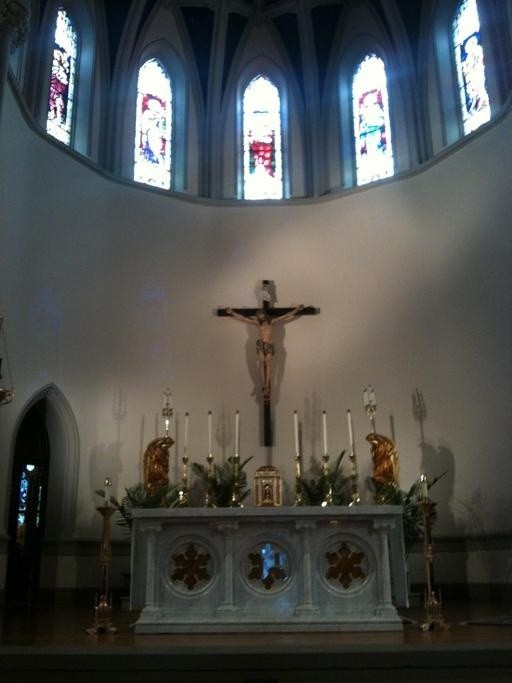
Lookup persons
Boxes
[226,303,304,395]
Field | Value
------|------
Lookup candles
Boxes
[290,408,354,456]
[179,407,243,458]
